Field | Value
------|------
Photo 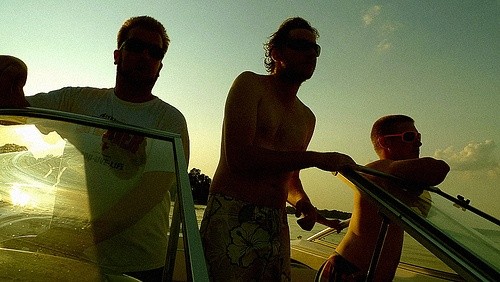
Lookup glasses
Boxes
[381,132,421,142]
[280,39,321,57]
[118,40,165,60]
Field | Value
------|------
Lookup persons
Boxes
[0,15,190,282]
[199,16,341,282]
[314,114,451,282]
